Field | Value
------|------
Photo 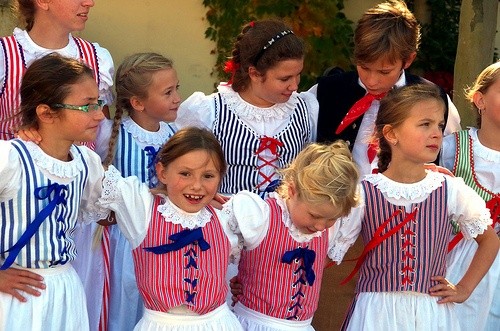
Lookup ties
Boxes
[335,91,389,164]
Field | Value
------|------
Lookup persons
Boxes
[1,52,230,331]
[316,0,463,183]
[176,20,319,202]
[93,52,183,331]
[0,0,115,153]
[15,125,246,331]
[325,83,500,331]
[225,140,454,331]
[228,82,500,331]
[437,59,500,331]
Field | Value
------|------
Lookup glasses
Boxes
[49,100,106,113]
